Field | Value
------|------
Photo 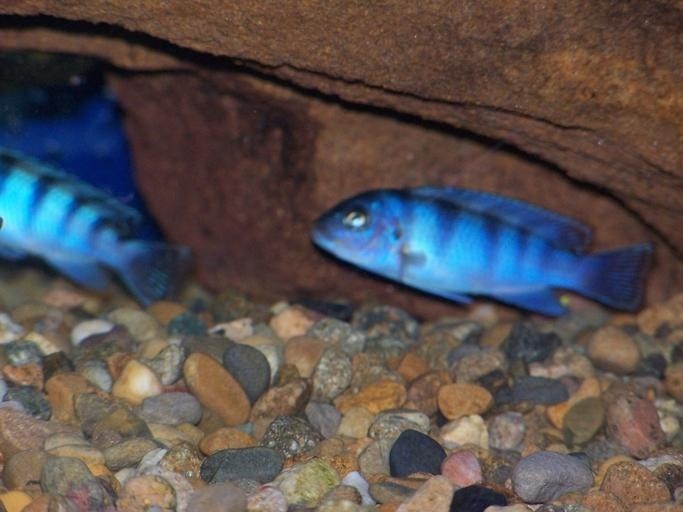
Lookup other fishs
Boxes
[0,145,195,309]
[309,182,656,319]
